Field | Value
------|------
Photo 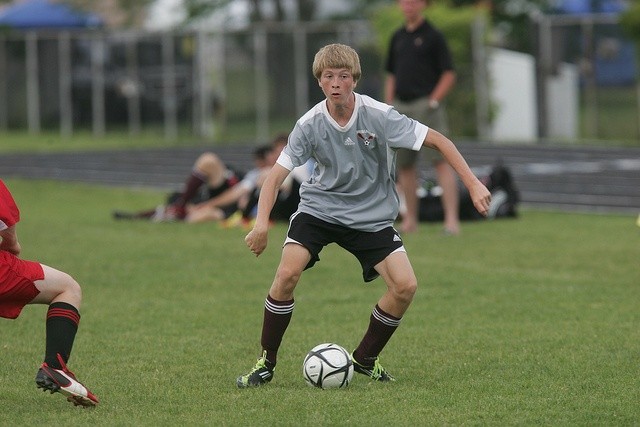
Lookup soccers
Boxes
[303,343,354,390]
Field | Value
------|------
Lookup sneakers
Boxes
[350,348,397,383]
[34,354,99,407]
[237,350,276,390]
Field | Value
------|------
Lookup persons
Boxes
[111,131,315,220]
[383,0,461,234]
[235,43,492,389]
[0,178,99,409]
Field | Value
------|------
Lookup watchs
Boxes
[428,99,441,110]
